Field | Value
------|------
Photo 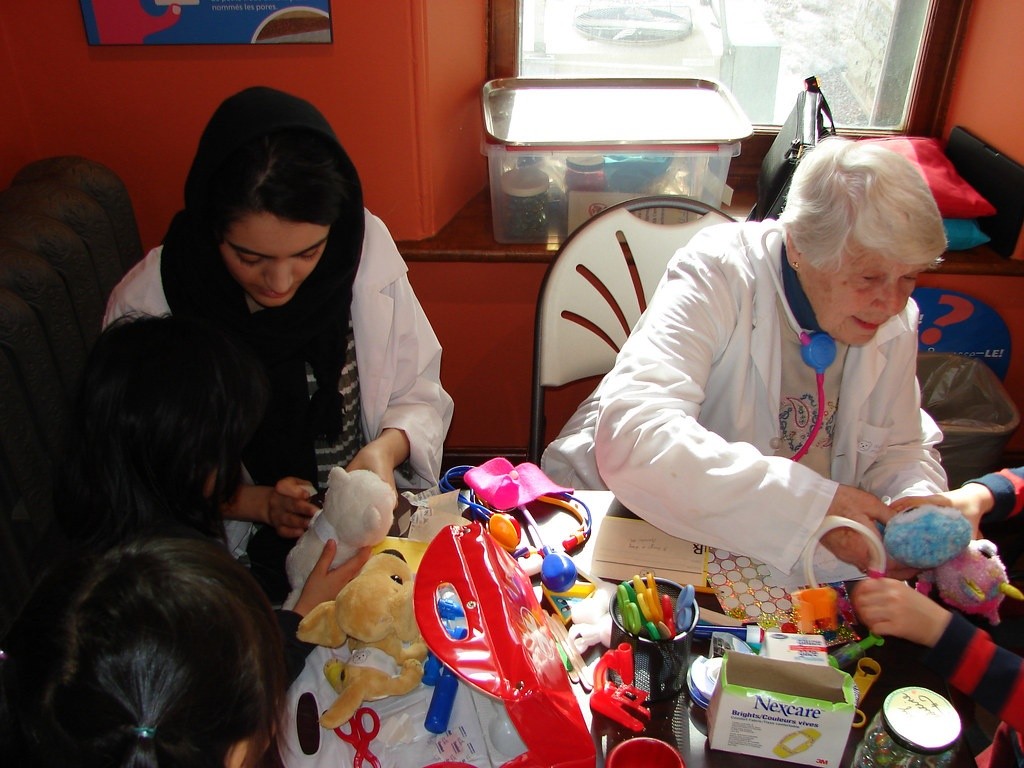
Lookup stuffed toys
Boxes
[281,467,397,611]
[295,550,429,730]
[874,494,1024,625]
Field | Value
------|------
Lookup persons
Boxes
[540,135,949,576]
[0,86,453,768]
[855,462,1024,768]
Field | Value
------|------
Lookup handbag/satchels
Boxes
[754,74,837,222]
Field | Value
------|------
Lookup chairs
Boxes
[529,197,738,466]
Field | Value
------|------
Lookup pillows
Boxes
[889,136,996,216]
[936,216,990,252]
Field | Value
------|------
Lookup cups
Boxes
[606,738,687,768]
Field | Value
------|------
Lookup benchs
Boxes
[0,156,145,767]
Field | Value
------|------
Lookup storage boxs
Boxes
[482,84,739,248]
[708,654,859,768]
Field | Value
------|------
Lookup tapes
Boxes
[803,515,888,590]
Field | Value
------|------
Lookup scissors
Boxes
[335,708,381,768]
[612,570,696,644]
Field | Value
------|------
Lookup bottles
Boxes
[500,154,605,239]
[850,686,964,768]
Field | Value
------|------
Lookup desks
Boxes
[290,491,978,768]
